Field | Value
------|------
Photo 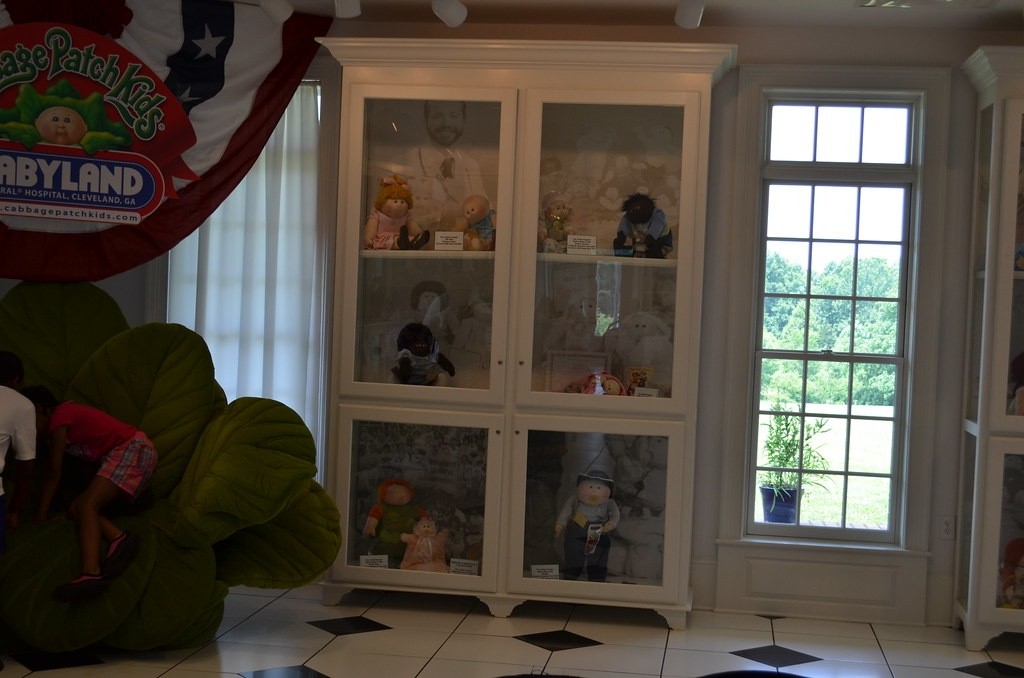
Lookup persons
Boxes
[389,99,493,224]
[23,386,156,604]
[0,351,38,577]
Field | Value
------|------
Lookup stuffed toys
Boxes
[999,538,1024,609]
[1002,454,1024,506]
[542,291,674,401]
[603,433,668,579]
[584,373,624,396]
[386,279,492,360]
[611,192,675,260]
[1006,351,1024,416]
[389,322,456,387]
[455,193,497,250]
[553,468,620,579]
[364,173,430,252]
[363,474,451,573]
[982,152,1024,271]
[537,191,578,253]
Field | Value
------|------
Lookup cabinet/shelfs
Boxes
[952,45,1024,653]
[313,38,738,632]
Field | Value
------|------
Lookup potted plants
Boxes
[758,381,836,523]
